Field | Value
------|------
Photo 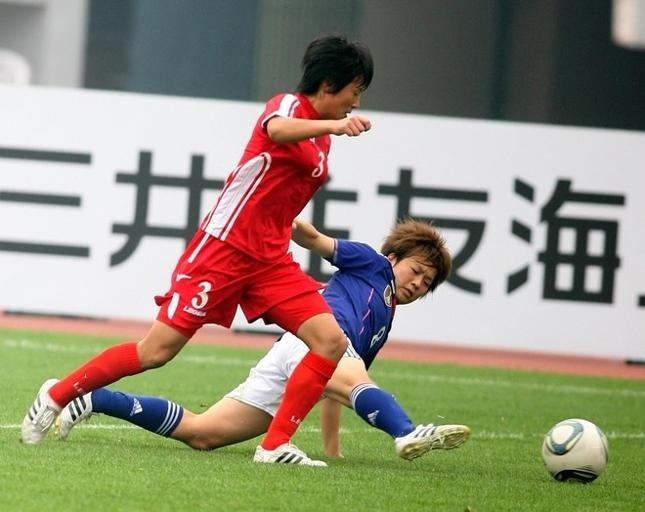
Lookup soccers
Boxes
[542,419,609,483]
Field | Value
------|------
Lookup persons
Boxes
[20,36,373,467]
[52,216,470,462]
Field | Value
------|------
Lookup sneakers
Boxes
[61,390,92,442]
[253,442,327,467]
[20,379,62,446]
[395,424,470,462]
